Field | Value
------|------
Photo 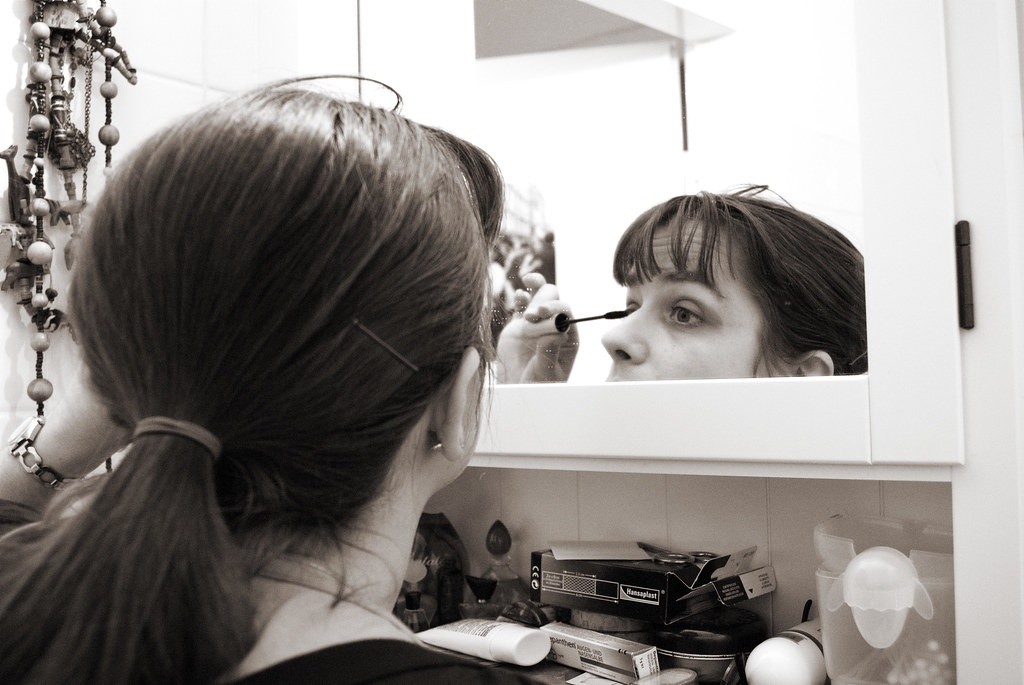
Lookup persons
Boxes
[1,74,551,685]
[496,181,866,384]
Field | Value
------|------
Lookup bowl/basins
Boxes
[638,629,751,681]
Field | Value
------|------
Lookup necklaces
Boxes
[266,548,391,610]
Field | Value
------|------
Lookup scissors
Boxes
[637,541,717,565]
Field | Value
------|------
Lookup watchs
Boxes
[6,415,82,494]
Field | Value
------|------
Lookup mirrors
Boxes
[356,0,963,468]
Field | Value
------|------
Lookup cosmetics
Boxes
[523,308,626,339]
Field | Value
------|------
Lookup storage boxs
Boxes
[539,620,660,685]
[530,545,778,626]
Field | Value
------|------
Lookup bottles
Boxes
[463,520,536,617]
